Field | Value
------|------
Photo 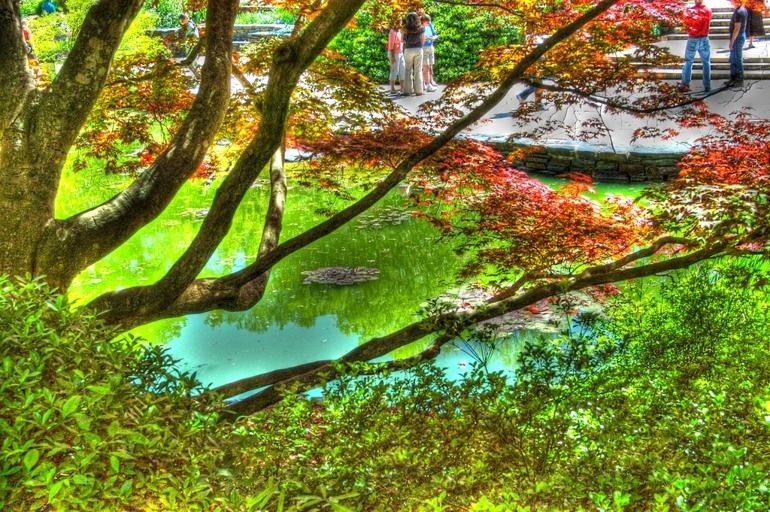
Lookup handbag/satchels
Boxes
[747,10,765,36]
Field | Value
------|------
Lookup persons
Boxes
[745,0,767,48]
[723,0,749,88]
[385,14,408,93]
[51,3,71,62]
[401,9,427,96]
[20,15,42,79]
[40,0,55,17]
[176,12,204,89]
[416,7,440,87]
[675,0,714,92]
[515,20,549,112]
[419,13,438,93]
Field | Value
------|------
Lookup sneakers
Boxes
[389,80,439,97]
[723,80,744,87]
